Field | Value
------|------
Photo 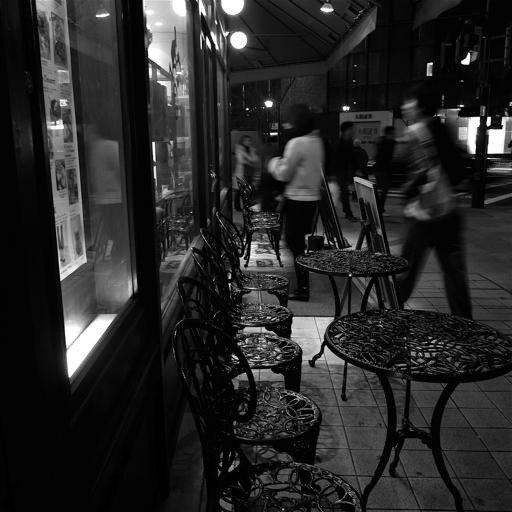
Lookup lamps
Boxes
[230,31,248,49]
[220,0,245,16]
[95,4,111,18]
[319,0,335,13]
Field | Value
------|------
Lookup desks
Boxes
[295,249,409,399]
[324,307,512,512]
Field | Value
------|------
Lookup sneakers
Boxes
[288,288,311,301]
[345,213,359,223]
[381,212,391,217]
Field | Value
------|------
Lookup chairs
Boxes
[198,227,294,340]
[170,317,364,512]
[175,276,323,466]
[214,210,290,308]
[232,174,284,267]
[190,246,302,392]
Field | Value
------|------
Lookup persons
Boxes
[329,121,360,223]
[391,84,474,320]
[353,137,369,178]
[231,133,259,211]
[267,102,326,302]
[372,125,396,218]
